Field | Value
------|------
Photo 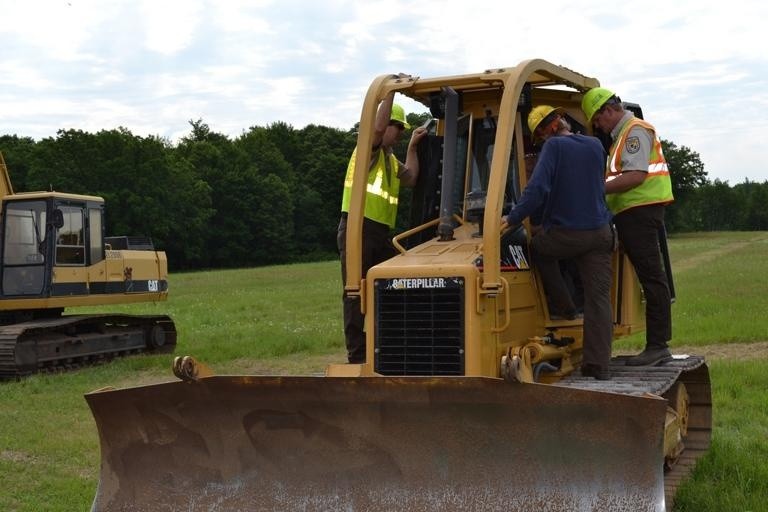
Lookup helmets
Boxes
[526,103,565,142]
[388,103,413,129]
[581,88,617,124]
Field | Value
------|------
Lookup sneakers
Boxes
[548,306,578,321]
[626,346,674,366]
[580,362,611,380]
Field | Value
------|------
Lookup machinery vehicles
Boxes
[86,60,715,511]
[1,152,178,380]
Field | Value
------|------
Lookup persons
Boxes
[581,87,674,367]
[500,104,614,381]
[337,87,428,365]
